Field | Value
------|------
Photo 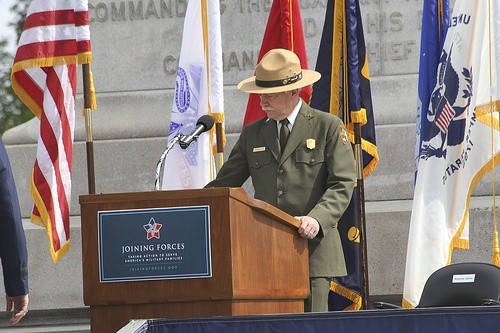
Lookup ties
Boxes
[279,118,291,152]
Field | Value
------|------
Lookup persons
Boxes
[205,49,356,312]
[0,138,30,326]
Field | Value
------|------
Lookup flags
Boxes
[414,0,453,189]
[163,1,228,191]
[11,0,95,263]
[242,0,309,129]
[308,0,380,311]
[401,0,500,311]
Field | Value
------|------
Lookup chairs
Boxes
[374,262,500,310]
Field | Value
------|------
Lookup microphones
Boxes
[179,115,214,149]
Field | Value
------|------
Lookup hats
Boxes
[236,48,321,94]
